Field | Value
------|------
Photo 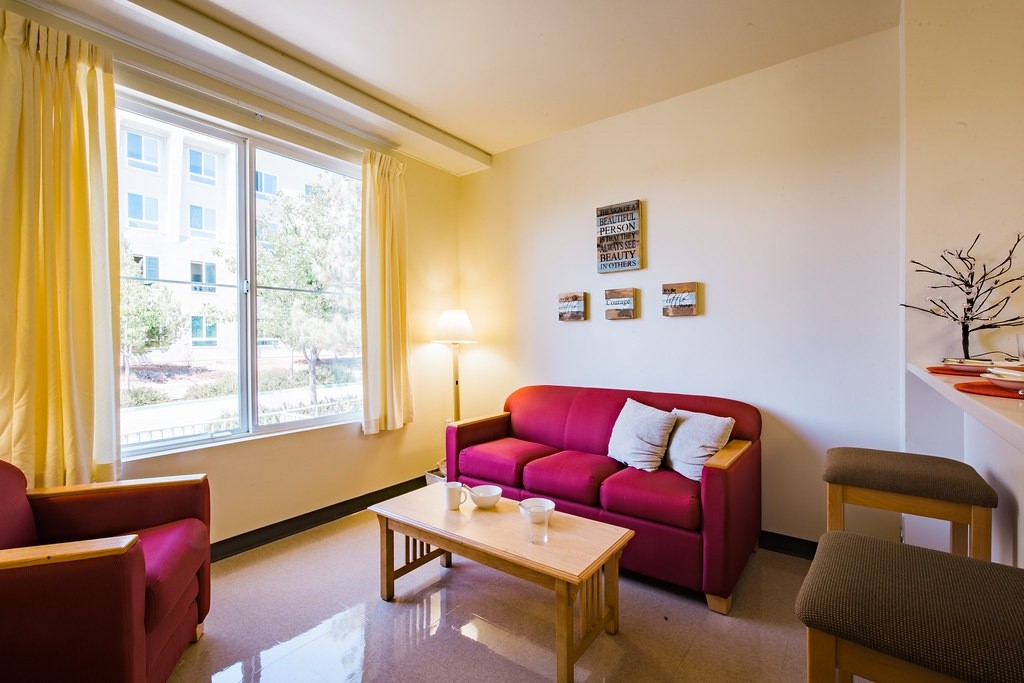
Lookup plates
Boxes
[980,373,1024,390]
[943,361,1024,371]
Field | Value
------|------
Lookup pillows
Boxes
[606,398,677,472]
[661,407,736,483]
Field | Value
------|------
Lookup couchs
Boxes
[446,386,762,616]
[0,458,211,683]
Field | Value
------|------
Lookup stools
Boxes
[823,447,998,561]
[794,532,1024,683]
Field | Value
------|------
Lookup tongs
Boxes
[986,367,1024,378]
[942,358,994,365]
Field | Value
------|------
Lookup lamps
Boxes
[428,309,477,421]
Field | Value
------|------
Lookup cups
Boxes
[444,482,467,510]
[1016,334,1024,362]
[526,506,549,545]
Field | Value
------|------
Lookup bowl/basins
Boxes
[470,485,502,508]
[519,498,555,523]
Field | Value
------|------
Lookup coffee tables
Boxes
[367,481,635,683]
